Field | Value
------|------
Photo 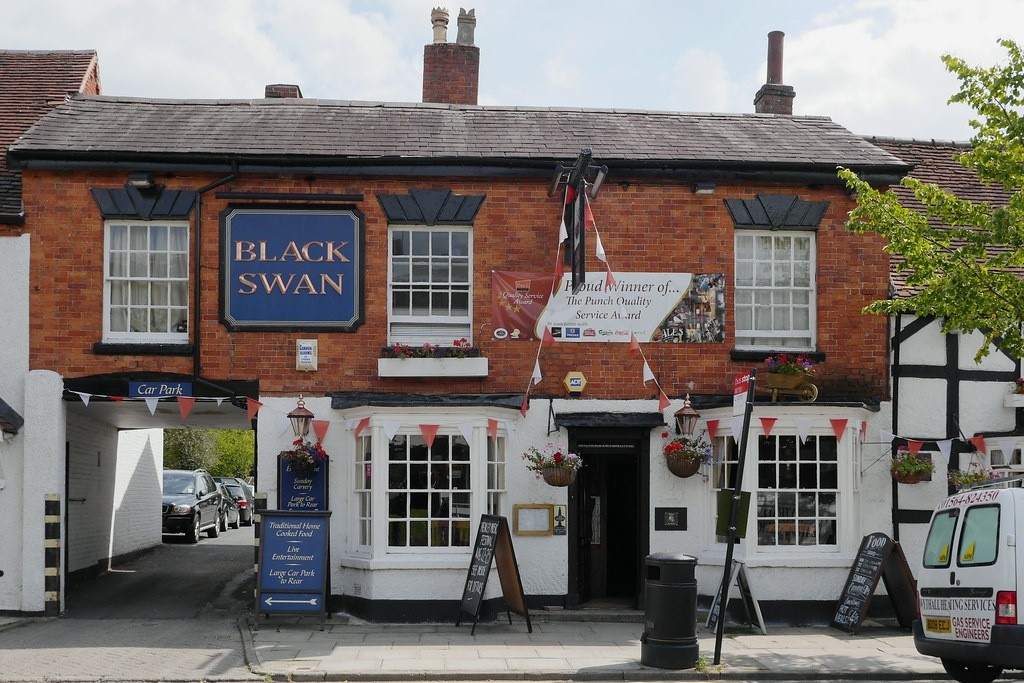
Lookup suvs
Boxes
[212,477,255,526]
[243,477,255,498]
[162,466,223,544]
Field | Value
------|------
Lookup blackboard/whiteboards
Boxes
[708,559,765,627]
[831,533,917,631]
[460,514,528,616]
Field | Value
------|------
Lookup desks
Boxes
[766,523,814,545]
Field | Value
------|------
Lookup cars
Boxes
[183,482,241,532]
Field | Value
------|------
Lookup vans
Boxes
[912,487,1024,683]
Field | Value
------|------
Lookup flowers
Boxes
[661,425,720,466]
[521,442,583,481]
[280,438,328,472]
[889,445,936,476]
[381,338,471,362]
[1008,377,1024,395]
[947,463,1001,490]
[763,352,817,377]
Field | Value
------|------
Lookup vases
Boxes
[763,372,806,389]
[541,466,574,486]
[893,470,925,484]
[378,357,489,377]
[1003,394,1024,407]
[287,464,317,477]
[666,458,700,478]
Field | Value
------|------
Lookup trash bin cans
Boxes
[642,552,699,670]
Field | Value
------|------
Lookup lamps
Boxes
[674,394,701,436]
[691,181,716,196]
[590,165,608,200]
[286,393,315,437]
[127,171,155,187]
[547,165,563,198]
